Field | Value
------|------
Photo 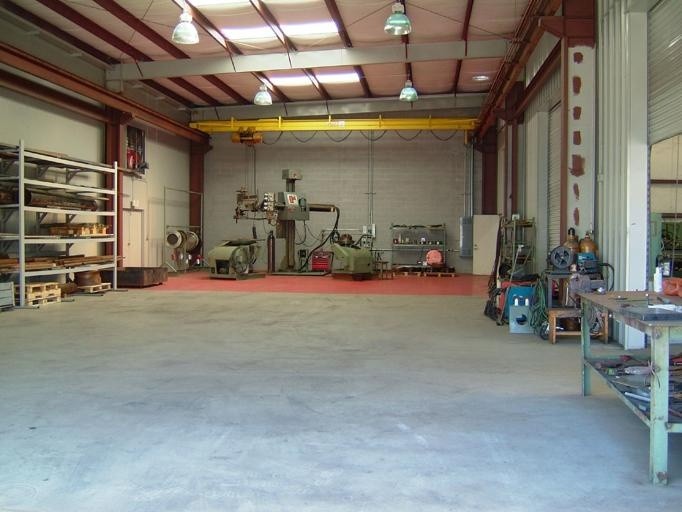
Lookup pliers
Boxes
[644,359,661,388]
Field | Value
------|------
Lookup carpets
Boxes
[122,270,489,296]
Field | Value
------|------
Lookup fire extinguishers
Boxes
[127,143,143,170]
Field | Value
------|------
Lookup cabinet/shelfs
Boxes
[390,224,446,272]
[0,139,129,310]
[501,216,536,275]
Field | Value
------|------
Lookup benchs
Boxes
[548,308,608,345]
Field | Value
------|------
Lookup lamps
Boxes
[384,0,412,35]
[171,0,200,45]
[253,83,273,105]
[398,71,419,103]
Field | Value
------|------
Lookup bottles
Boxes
[654,267,662,293]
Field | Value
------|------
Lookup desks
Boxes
[576,290,682,487]
